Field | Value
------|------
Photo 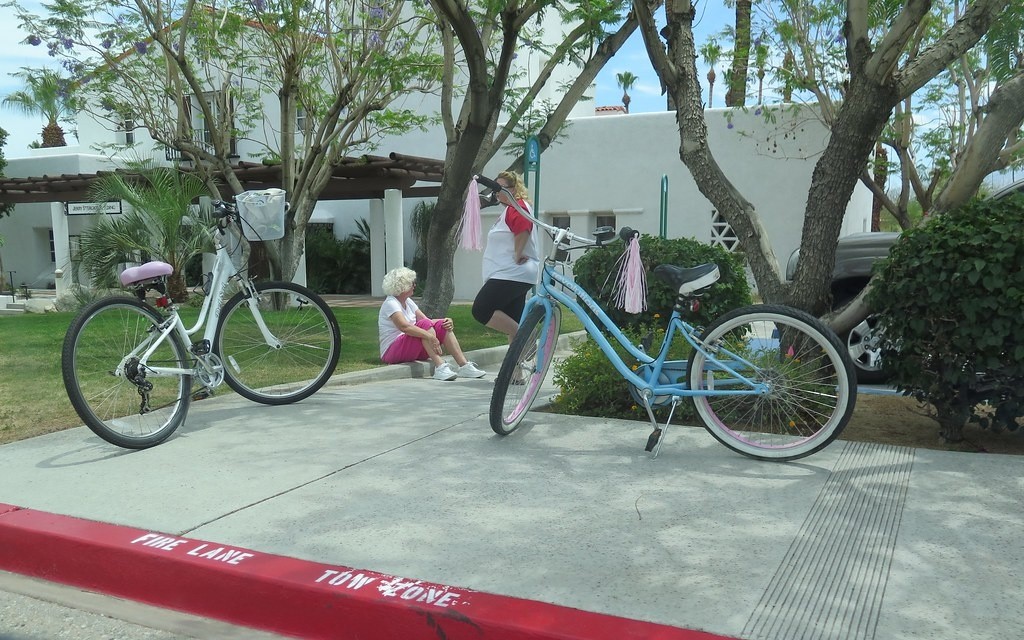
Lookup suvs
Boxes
[788,179,1024,384]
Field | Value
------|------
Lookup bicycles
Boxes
[454,175,858,462]
[61,188,342,450]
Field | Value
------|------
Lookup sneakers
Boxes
[494,376,527,386]
[525,327,537,361]
[433,362,457,381]
[454,361,486,378]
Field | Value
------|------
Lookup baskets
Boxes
[235,188,287,241]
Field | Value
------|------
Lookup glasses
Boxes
[501,184,514,189]
[412,281,415,286]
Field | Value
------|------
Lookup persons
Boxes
[471,172,541,385]
[377,267,486,381]
